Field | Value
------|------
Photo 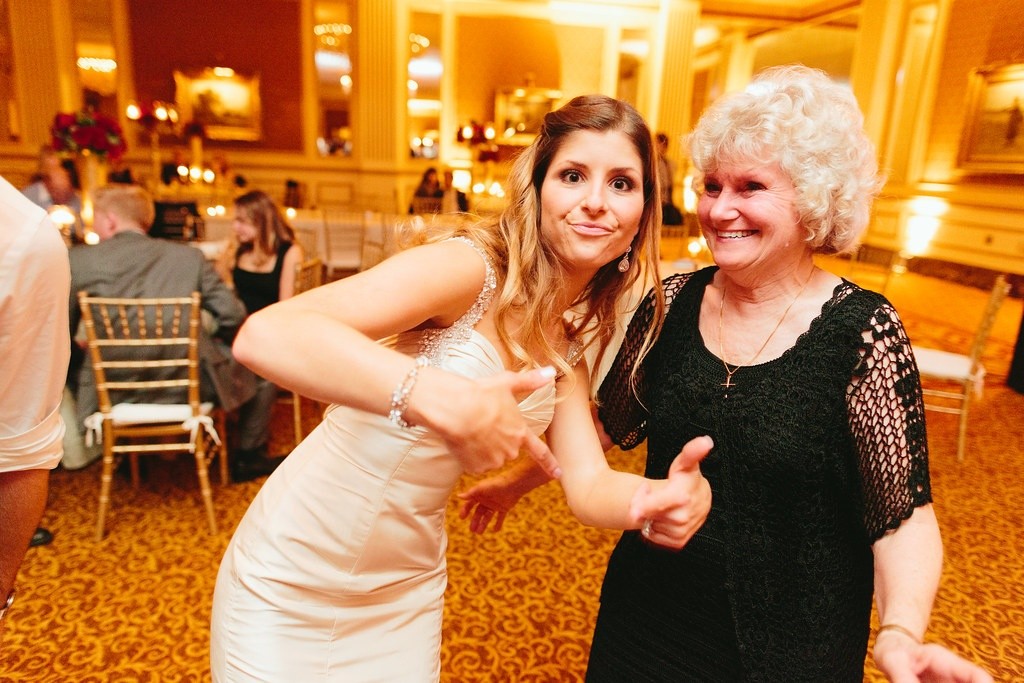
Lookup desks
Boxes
[280,211,462,268]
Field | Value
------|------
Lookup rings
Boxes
[644,520,654,534]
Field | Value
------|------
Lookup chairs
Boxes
[911,272,1010,458]
[79,291,227,538]
[266,210,387,447]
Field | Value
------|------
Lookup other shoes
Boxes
[233,455,287,482]
[120,457,150,479]
[29,528,50,546]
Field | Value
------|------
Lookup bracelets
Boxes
[876,624,920,645]
[389,359,430,428]
[0,590,17,620]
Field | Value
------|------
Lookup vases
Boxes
[73,152,107,190]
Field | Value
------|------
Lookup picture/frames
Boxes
[173,68,261,141]
[954,63,1024,177]
[495,89,561,146]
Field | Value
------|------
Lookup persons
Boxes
[211,95,714,683]
[409,167,469,213]
[27,175,300,478]
[0,174,71,625]
[657,134,673,204]
[457,68,994,683]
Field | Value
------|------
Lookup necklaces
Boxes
[719,264,817,397]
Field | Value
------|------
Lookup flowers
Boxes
[46,109,128,164]
